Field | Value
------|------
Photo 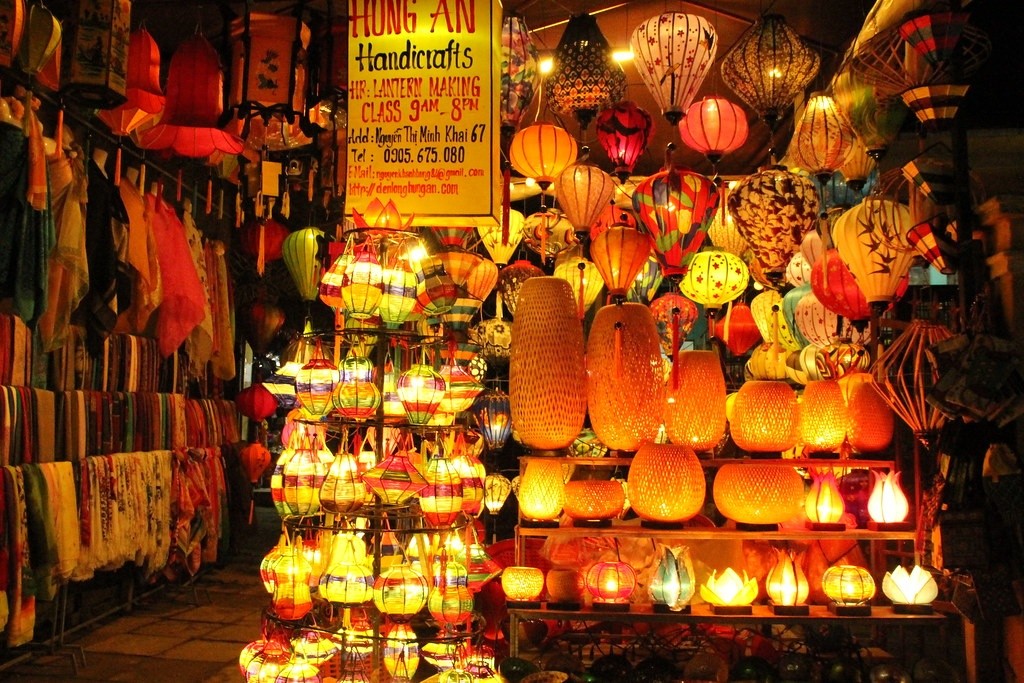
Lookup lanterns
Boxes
[1,1,1024,683]
